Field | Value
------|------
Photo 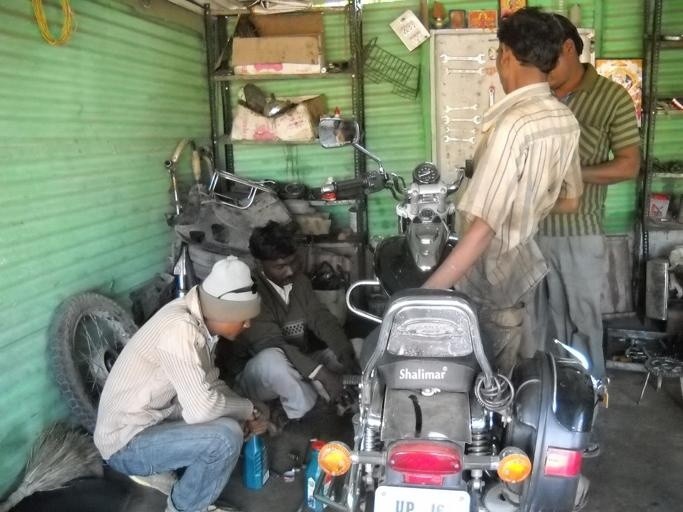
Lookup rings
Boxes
[342,384,348,389]
[343,394,349,399]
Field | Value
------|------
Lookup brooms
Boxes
[0,420,111,512]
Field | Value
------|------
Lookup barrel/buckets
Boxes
[242,434,269,492]
[305,442,336,512]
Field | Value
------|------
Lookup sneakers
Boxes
[128,472,179,496]
[162,494,240,511]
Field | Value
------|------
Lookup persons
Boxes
[92,256,271,511]
[418,2,586,371]
[532,14,642,460]
[221,224,366,428]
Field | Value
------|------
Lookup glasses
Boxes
[217,273,257,302]
[486,46,501,62]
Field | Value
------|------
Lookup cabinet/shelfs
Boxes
[634,2,682,331]
[202,0,370,322]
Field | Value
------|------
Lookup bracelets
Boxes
[250,406,260,418]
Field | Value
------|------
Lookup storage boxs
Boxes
[228,93,326,144]
[214,10,326,75]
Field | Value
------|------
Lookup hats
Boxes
[197,253,263,324]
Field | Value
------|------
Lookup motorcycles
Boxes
[304,114,612,512]
